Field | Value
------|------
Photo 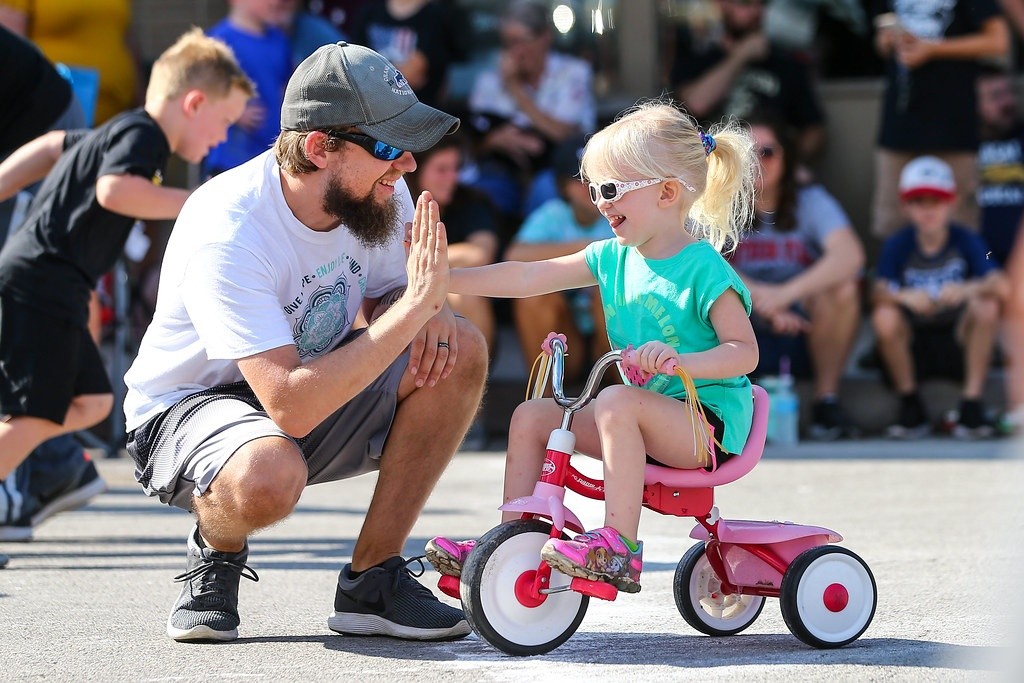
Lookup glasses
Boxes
[321,128,404,161]
[589,177,696,208]
[754,147,779,158]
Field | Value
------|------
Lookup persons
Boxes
[189,0,354,195]
[0,28,260,565]
[861,0,1023,218]
[448,101,762,601]
[996,200,1023,438]
[401,126,497,376]
[502,130,630,426]
[703,113,867,441]
[660,0,831,221]
[121,40,489,641]
[1,18,109,543]
[321,0,469,108]
[470,0,600,203]
[869,156,1009,442]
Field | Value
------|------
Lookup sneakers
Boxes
[167,521,259,640]
[31,440,107,526]
[424,536,477,577]
[540,525,643,593]
[327,555,472,640]
[0,478,32,541]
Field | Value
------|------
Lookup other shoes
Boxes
[954,401,994,439]
[889,397,933,438]
[808,399,843,438]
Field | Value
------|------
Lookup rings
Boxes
[438,342,450,348]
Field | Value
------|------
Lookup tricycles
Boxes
[436,331,878,658]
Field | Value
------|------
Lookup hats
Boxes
[898,155,957,202]
[280,41,461,152]
